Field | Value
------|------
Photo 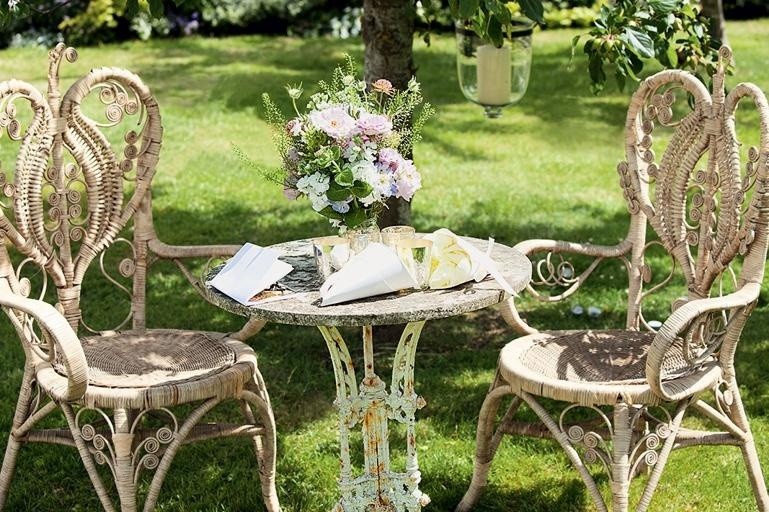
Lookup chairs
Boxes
[457,41,767,512]
[0,41,291,512]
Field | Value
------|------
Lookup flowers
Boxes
[311,226,521,310]
[225,50,439,222]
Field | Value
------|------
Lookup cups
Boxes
[380,226,433,291]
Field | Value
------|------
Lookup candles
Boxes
[456,16,536,119]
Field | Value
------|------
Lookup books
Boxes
[212,242,299,308]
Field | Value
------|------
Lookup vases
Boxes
[336,209,383,262]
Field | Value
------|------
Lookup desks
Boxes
[201,228,532,512]
